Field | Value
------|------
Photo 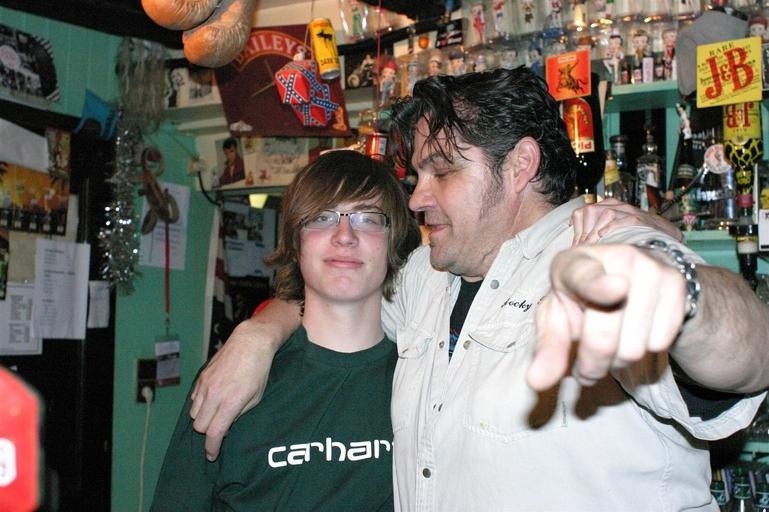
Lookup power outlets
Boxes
[135,358,158,403]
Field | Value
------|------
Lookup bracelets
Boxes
[628,238,701,343]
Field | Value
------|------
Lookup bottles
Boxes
[713,457,769,512]
[636,121,668,214]
[735,192,759,256]
[665,116,735,220]
[722,100,765,183]
[603,133,634,206]
[565,70,603,205]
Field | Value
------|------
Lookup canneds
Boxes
[306,18,341,80]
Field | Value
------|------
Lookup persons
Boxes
[149,150,398,512]
[189,63,769,512]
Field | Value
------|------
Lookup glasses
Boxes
[301,207,388,236]
